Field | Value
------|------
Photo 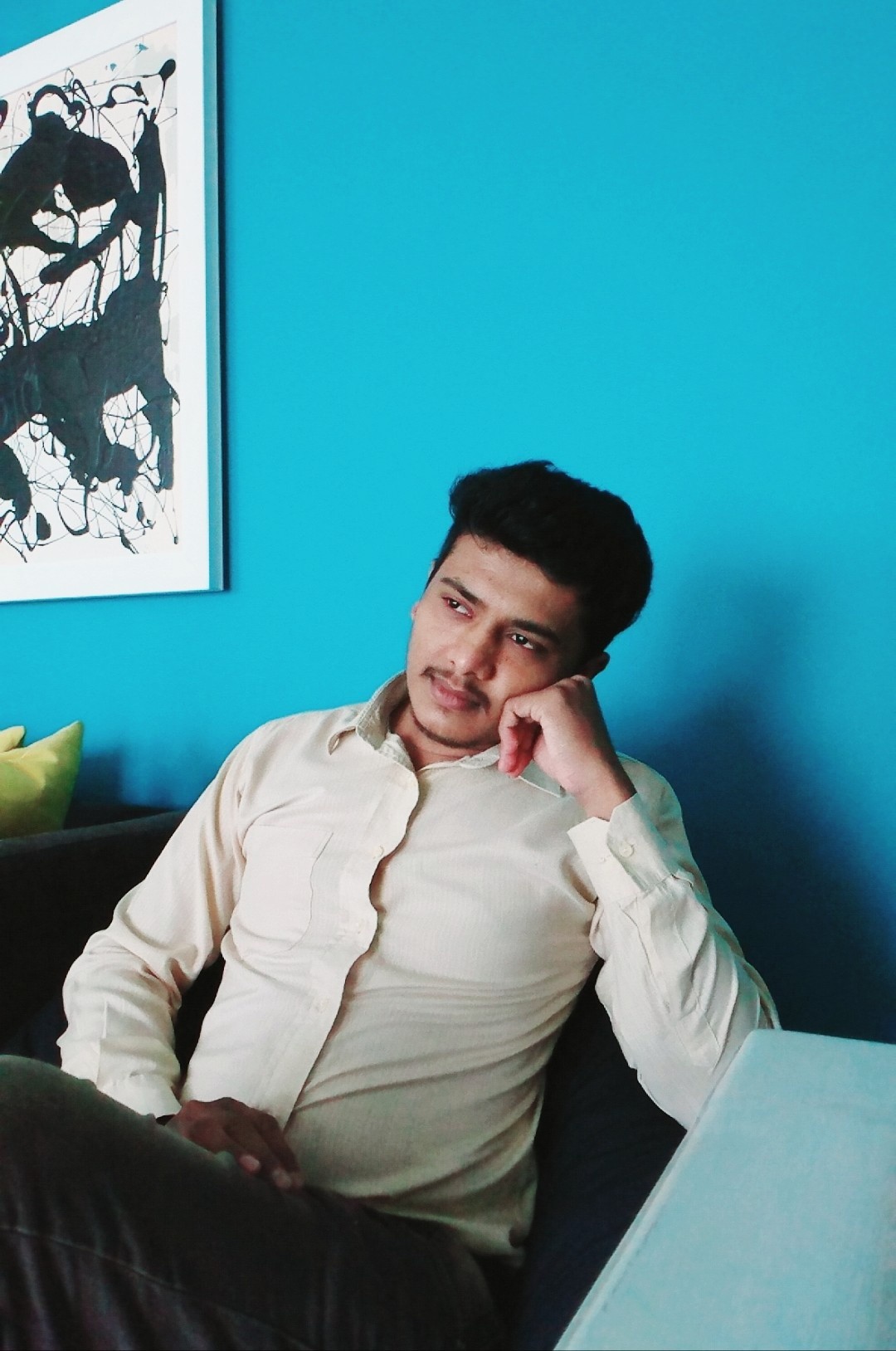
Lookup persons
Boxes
[0,459,784,1350]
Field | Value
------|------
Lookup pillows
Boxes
[0,719,84,840]
